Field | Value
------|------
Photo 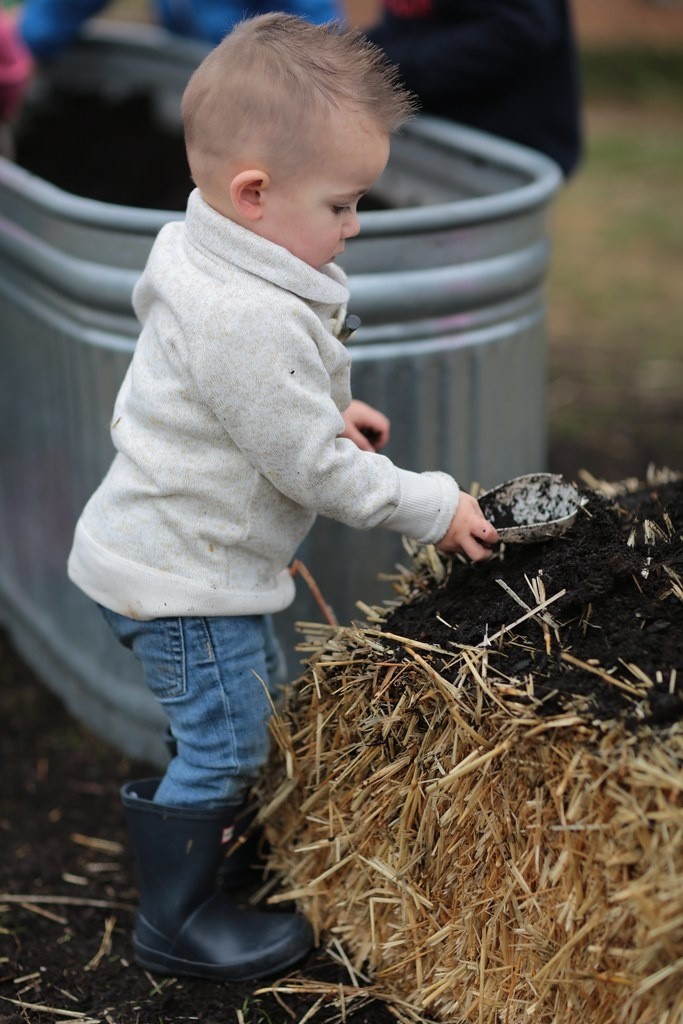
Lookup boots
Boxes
[120,778,314,980]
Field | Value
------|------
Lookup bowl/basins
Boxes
[477,471,581,544]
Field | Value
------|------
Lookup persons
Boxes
[67,13,502,985]
[20,0,342,54]
[358,0,582,176]
